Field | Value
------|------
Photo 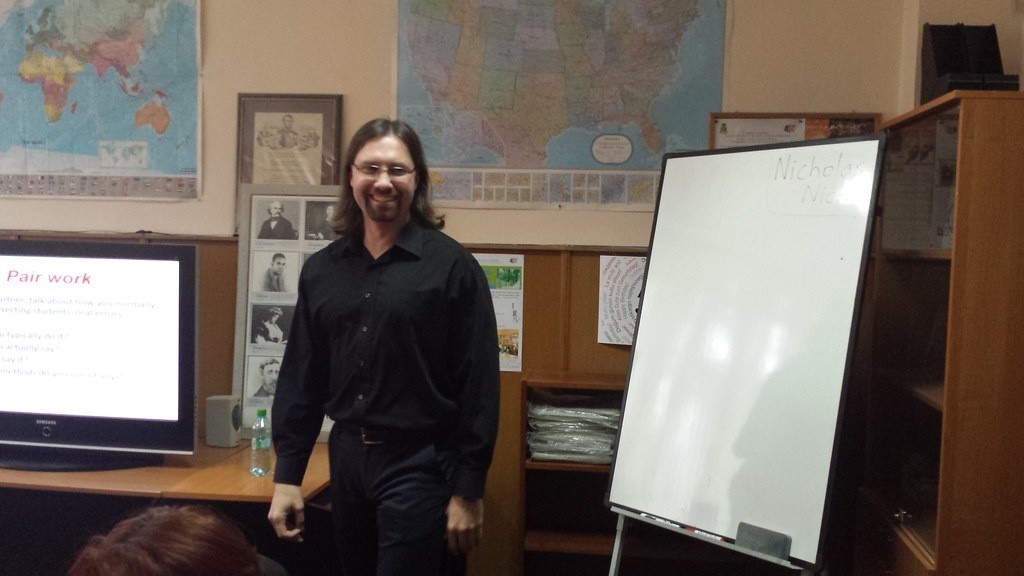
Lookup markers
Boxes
[685,527,724,542]
[640,513,681,528]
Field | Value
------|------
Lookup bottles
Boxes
[250,407,271,477]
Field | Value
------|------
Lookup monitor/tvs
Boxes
[0,239,198,473]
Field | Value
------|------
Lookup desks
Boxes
[0,440,331,506]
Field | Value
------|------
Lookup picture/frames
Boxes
[233,90,344,235]
[707,110,884,153]
[230,182,344,442]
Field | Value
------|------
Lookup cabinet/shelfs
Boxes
[854,90,1024,576]
[513,371,801,576]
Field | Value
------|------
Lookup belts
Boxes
[334,421,453,446]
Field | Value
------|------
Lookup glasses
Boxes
[353,159,417,183]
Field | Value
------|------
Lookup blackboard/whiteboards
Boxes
[603,132,888,573]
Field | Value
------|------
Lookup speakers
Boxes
[205,394,242,447]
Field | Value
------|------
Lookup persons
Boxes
[267,117,502,576]
[66,505,262,576]
[258,200,296,239]
[314,205,336,240]
[254,307,284,344]
[252,358,280,398]
[262,252,288,293]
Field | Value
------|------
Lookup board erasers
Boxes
[734,522,792,562]
